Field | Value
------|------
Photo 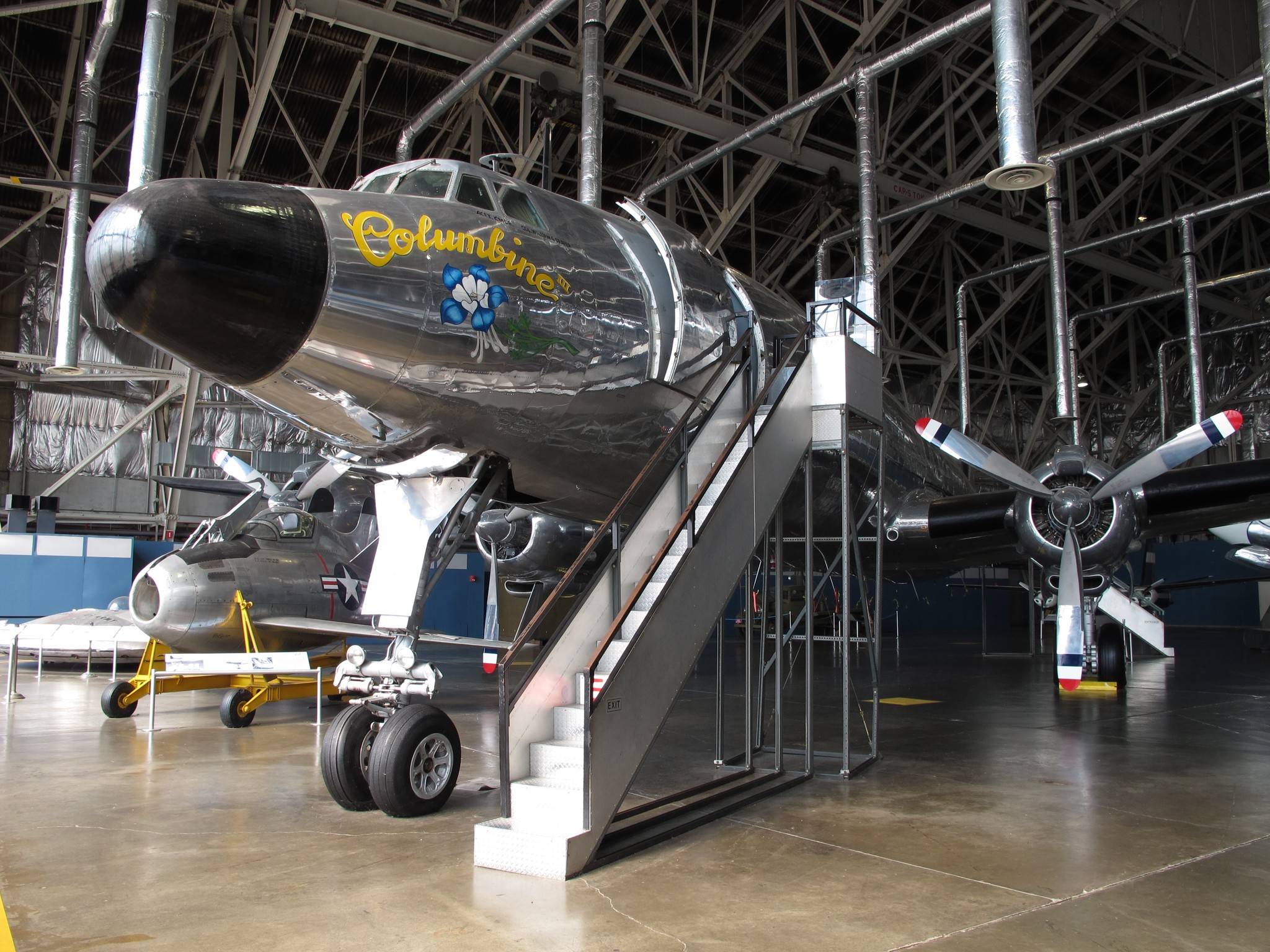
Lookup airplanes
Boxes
[101,451,377,730]
[77,156,1249,822]
